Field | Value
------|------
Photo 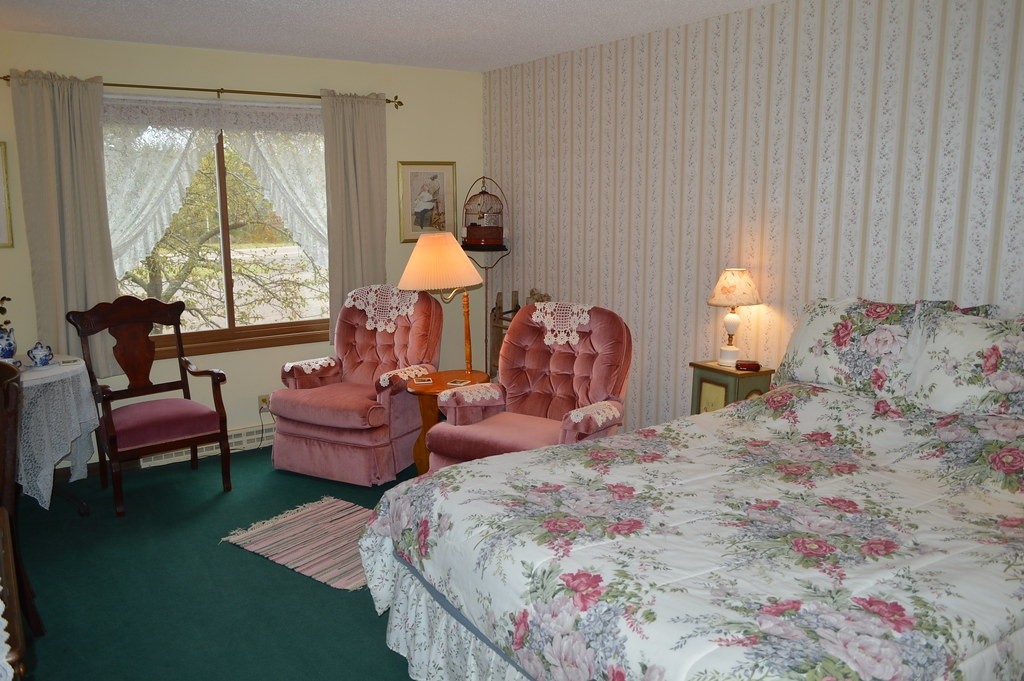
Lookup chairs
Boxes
[0,361,47,638]
[424,300,634,473]
[65,295,233,518]
[268,284,444,488]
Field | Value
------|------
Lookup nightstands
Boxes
[687,358,776,416]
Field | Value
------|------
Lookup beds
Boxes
[359,383,1024,681]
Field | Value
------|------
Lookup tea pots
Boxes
[0,327,17,361]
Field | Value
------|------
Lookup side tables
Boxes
[405,369,491,478]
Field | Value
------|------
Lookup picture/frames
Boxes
[396,160,459,245]
[0,141,15,249]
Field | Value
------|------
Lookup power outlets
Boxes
[258,395,270,413]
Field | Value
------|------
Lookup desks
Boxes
[0,352,99,519]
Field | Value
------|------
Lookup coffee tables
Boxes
[0,507,34,681]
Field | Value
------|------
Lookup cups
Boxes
[32,343,53,367]
[0,358,23,369]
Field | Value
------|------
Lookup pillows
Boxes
[768,296,921,400]
[872,297,1024,424]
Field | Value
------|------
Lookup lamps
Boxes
[397,231,484,373]
[705,268,765,368]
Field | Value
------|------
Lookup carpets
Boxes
[218,496,376,593]
